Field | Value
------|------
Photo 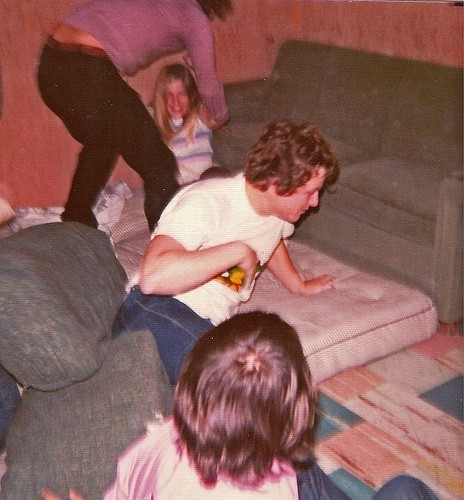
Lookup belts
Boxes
[46,36,104,58]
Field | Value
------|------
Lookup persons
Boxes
[291,454,438,500]
[37,0,230,233]
[145,62,216,182]
[112,120,337,392]
[42,311,316,500]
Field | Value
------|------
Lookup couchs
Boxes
[212,38,463,323]
